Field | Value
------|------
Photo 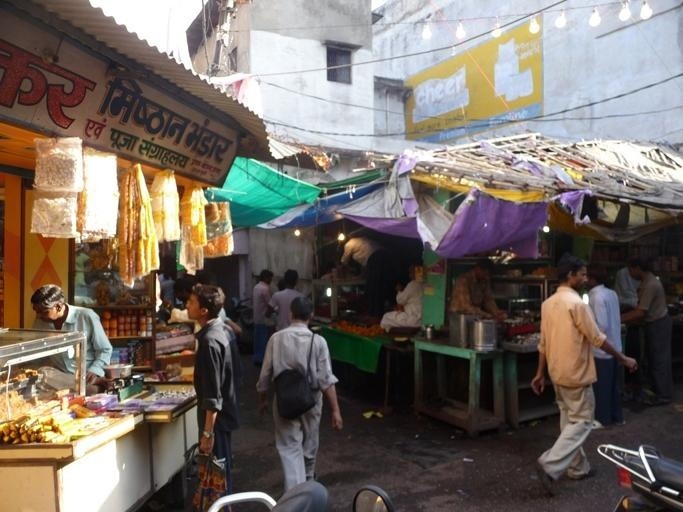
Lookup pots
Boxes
[446,310,499,352]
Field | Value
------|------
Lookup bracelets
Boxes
[202,431,215,437]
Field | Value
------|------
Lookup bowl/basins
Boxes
[102,363,133,380]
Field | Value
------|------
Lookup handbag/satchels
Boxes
[272,369,317,417]
[183,444,228,511]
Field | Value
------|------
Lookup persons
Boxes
[255,295,343,494]
[581,249,673,429]
[24,283,112,389]
[529,250,641,497]
[183,282,238,512]
[334,231,393,319]
[378,263,423,332]
[448,258,509,322]
[154,256,311,368]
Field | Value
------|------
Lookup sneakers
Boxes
[533,458,558,499]
[589,421,610,429]
[572,465,600,480]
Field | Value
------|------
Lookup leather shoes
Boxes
[642,396,673,406]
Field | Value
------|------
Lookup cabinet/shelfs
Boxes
[0,410,154,512]
[85,304,154,372]
[117,377,199,495]
[498,335,558,430]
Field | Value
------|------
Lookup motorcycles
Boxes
[596,444,682,512]
[205,483,397,512]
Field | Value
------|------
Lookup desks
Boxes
[316,319,410,413]
[411,333,505,442]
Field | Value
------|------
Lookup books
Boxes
[82,393,183,413]
